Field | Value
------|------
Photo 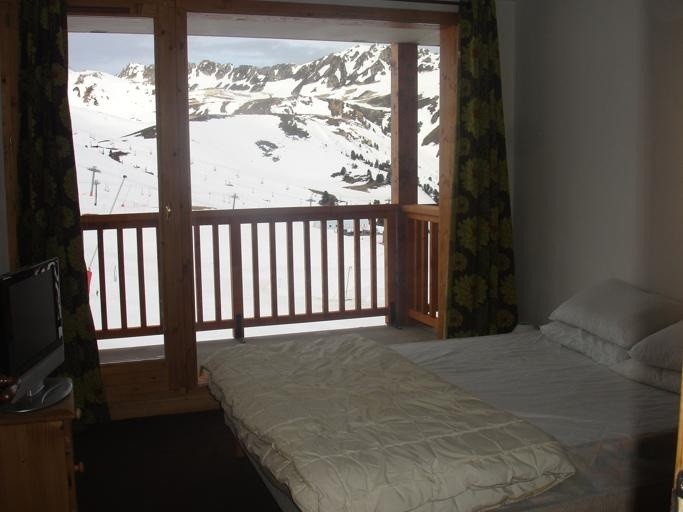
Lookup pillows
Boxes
[539,277,683,397]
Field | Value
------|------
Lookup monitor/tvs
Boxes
[0,258,73,414]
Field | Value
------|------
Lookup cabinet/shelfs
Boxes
[0,387,84,512]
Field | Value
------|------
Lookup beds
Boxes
[200,331,683,511]
